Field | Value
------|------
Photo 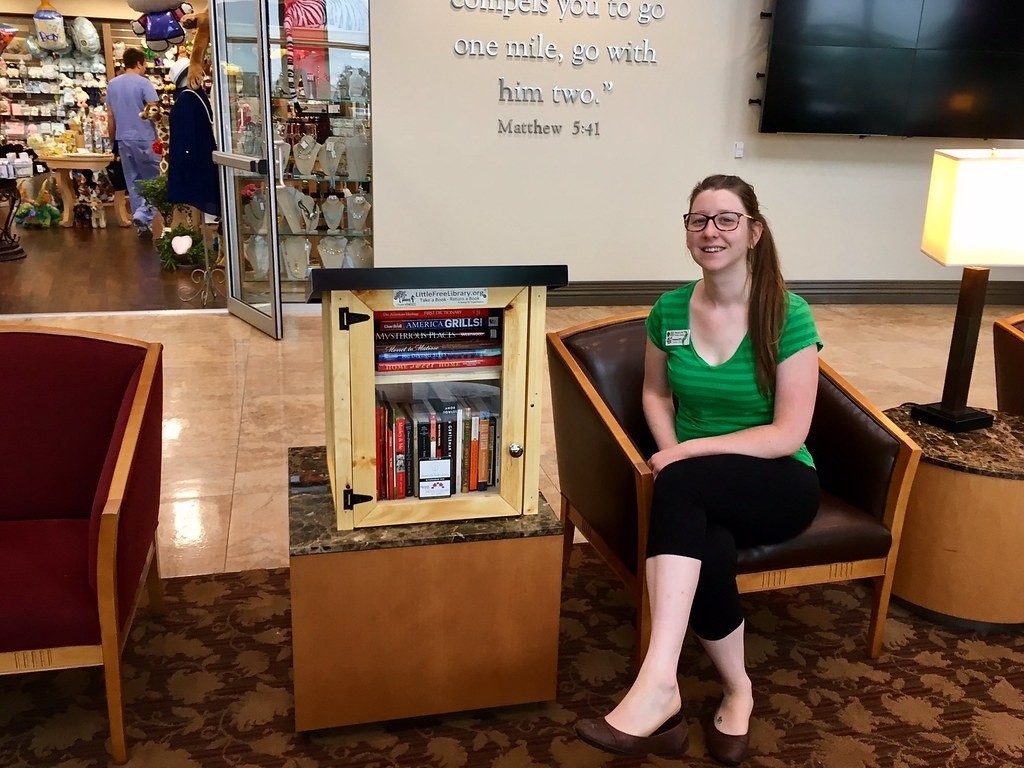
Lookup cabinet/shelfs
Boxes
[0,14,202,153]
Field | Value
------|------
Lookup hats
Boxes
[169,58,190,86]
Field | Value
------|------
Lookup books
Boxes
[373,311,502,496]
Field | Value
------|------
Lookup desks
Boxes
[38,154,137,228]
[876,400,1024,632]
[284,443,567,735]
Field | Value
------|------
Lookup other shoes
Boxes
[573,716,689,756]
[134,217,152,242]
[710,712,751,763]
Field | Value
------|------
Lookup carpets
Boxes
[0,540,1024,768]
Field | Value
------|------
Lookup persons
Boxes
[105,48,162,239]
[574,172,824,768]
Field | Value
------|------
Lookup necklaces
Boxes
[235,115,374,282]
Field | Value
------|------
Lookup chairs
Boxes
[992,310,1024,422]
[547,309,924,684]
[0,323,163,762]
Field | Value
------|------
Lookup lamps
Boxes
[901,149,1024,430]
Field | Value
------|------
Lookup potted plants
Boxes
[159,224,215,271]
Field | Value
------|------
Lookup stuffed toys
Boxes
[0,0,213,228]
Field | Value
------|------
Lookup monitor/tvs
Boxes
[759,0,1024,140]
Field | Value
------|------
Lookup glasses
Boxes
[684,211,756,232]
